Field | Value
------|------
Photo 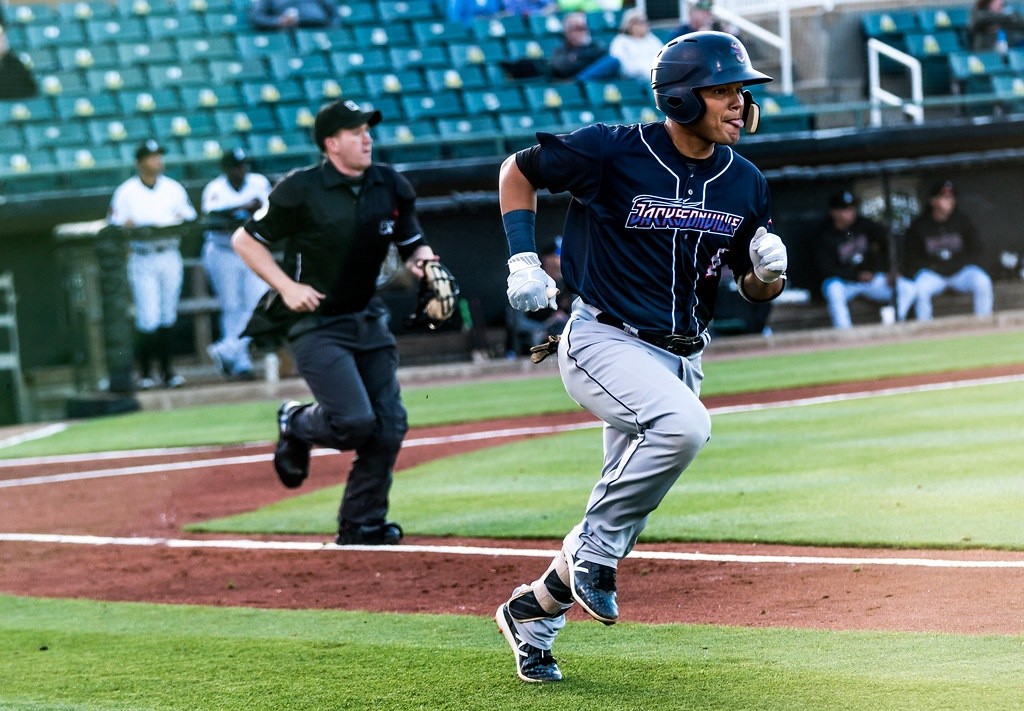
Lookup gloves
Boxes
[508,252,559,312]
[530,335,559,364]
[749,227,787,283]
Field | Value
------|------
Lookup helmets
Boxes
[650,30,773,126]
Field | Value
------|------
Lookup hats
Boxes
[829,189,860,210]
[314,99,383,144]
[565,12,587,29]
[928,179,955,198]
[222,146,247,168]
[691,0,714,10]
[135,140,168,161]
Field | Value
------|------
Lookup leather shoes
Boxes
[273,401,310,488]
[335,522,403,545]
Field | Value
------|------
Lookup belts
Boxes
[596,312,708,357]
[136,245,167,256]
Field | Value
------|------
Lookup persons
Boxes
[961,0,1024,55]
[0,24,34,98]
[555,0,623,13]
[446,297,487,364]
[896,177,994,319]
[252,0,337,28]
[823,192,894,326]
[555,12,618,85]
[447,0,558,22]
[494,30,789,686]
[107,140,197,388]
[201,147,274,381]
[609,10,665,78]
[230,99,457,544]
[717,269,773,335]
[512,234,573,346]
[668,0,744,43]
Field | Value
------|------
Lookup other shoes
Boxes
[139,376,156,389]
[206,344,234,381]
[234,368,258,382]
[161,371,185,387]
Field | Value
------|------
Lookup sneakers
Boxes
[563,546,619,626]
[493,602,563,682]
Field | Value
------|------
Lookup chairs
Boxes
[856,0,1024,114]
[0,0,816,176]
